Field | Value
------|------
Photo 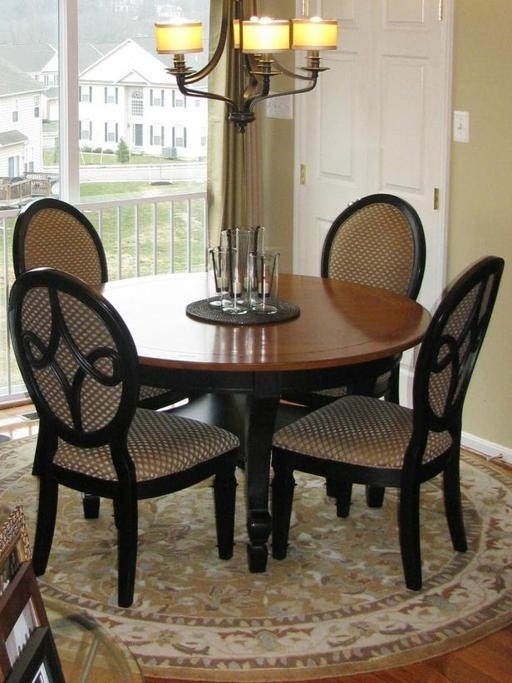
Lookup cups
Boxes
[210,226,279,315]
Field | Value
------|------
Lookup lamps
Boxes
[154,0,338,135]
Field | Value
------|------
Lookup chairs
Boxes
[7,266,247,606]
[278,192,429,521]
[267,251,505,595]
[13,196,190,520]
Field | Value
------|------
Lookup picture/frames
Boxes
[0,505,67,682]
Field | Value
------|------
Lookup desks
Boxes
[83,266,435,578]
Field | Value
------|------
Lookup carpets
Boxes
[0,432,512,682]
